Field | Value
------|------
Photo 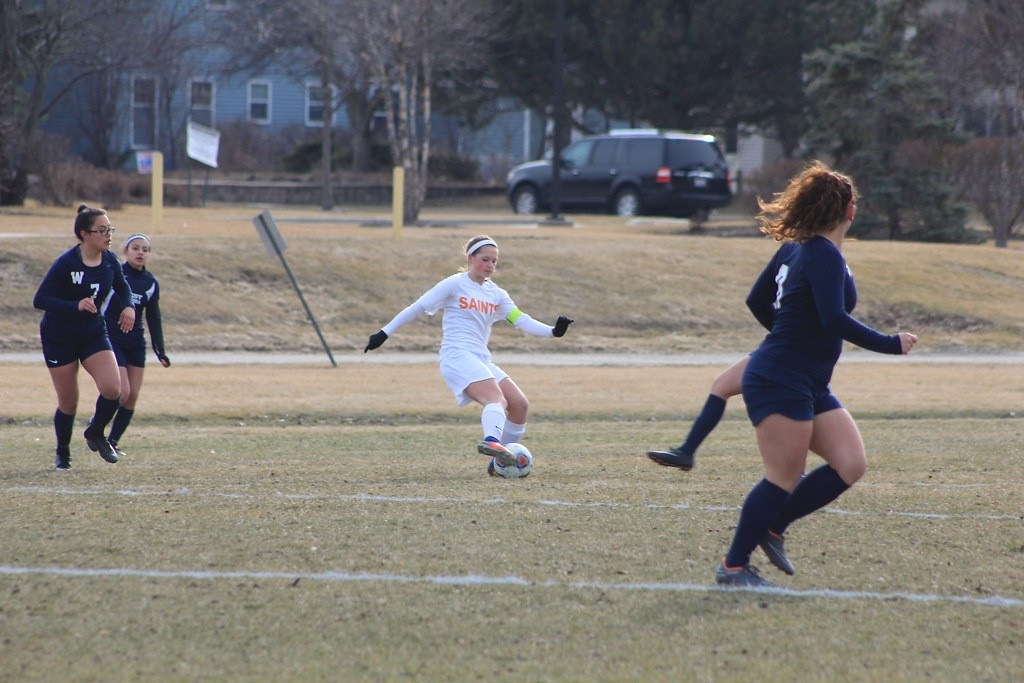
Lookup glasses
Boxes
[89,227,116,236]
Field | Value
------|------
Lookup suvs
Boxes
[504,128,733,222]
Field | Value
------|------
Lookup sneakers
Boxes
[646,447,693,470]
[108,438,126,457]
[764,529,794,575]
[715,557,773,588]
[487,459,497,476]
[478,440,516,464]
[55,446,71,471]
[84,426,117,465]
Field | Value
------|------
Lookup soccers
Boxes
[493,443,533,479]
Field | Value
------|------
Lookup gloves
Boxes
[553,316,574,337]
[364,330,387,354]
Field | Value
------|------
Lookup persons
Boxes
[712,157,921,588]
[363,234,575,478]
[32,202,136,472]
[647,346,760,472]
[85,232,171,457]
